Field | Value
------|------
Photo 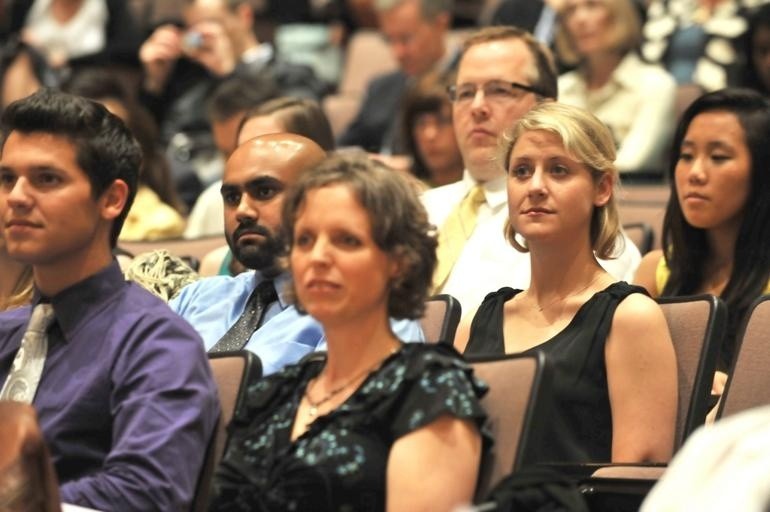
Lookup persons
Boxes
[167,135,425,375]
[454,104,678,512]
[0,1,769,214]
[1,89,222,510]
[418,28,640,321]
[637,89,770,302]
[213,147,493,512]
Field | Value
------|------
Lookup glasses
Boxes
[446,80,538,102]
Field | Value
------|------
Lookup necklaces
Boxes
[524,270,602,313]
[303,355,390,414]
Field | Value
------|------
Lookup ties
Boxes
[0,302,55,404]
[428,186,487,300]
[207,280,276,353]
